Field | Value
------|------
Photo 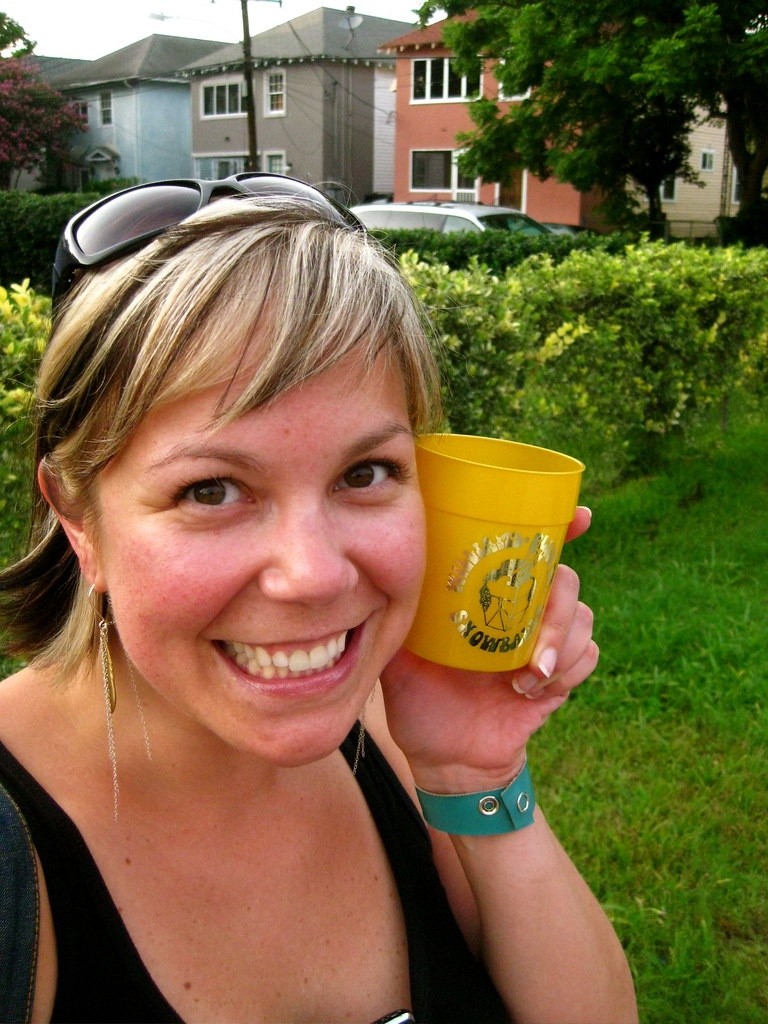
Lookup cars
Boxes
[513,223,599,238]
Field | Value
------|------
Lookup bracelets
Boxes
[414,756,538,840]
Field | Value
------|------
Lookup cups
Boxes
[405,435,584,678]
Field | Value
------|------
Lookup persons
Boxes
[0,174,639,1024]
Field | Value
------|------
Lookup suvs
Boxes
[348,199,554,238]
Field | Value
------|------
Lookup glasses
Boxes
[51,172,368,325]
